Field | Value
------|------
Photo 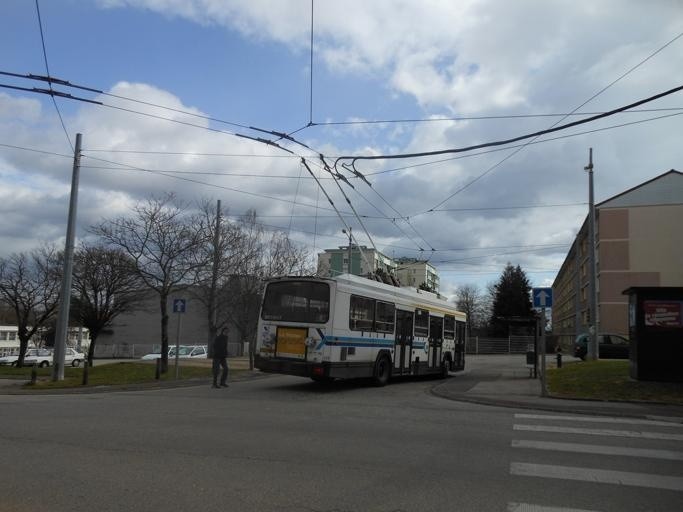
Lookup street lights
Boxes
[342,227,352,274]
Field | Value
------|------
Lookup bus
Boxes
[254,274,467,387]
[0,326,91,348]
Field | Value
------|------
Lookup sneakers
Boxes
[211,383,229,389]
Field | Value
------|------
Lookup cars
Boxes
[0,348,85,368]
[574,333,629,359]
[141,345,208,361]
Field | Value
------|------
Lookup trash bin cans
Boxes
[526,351,535,364]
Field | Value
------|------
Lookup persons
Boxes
[211,325,228,390]
